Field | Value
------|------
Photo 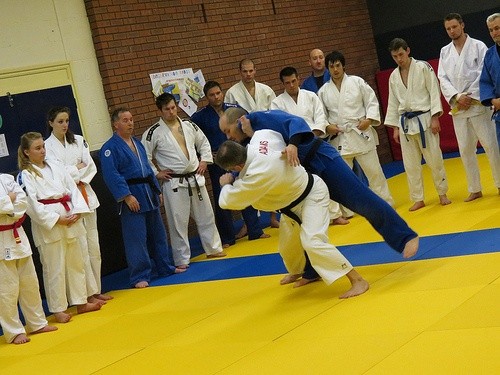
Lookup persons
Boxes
[317,51,393,220]
[437,13,500,202]
[219,108,419,288]
[190,59,350,247]
[216,116,369,298]
[139,93,226,268]
[300,49,331,93]
[383,39,452,211]
[98,108,187,288]
[0,106,114,344]
[479,13,500,146]
